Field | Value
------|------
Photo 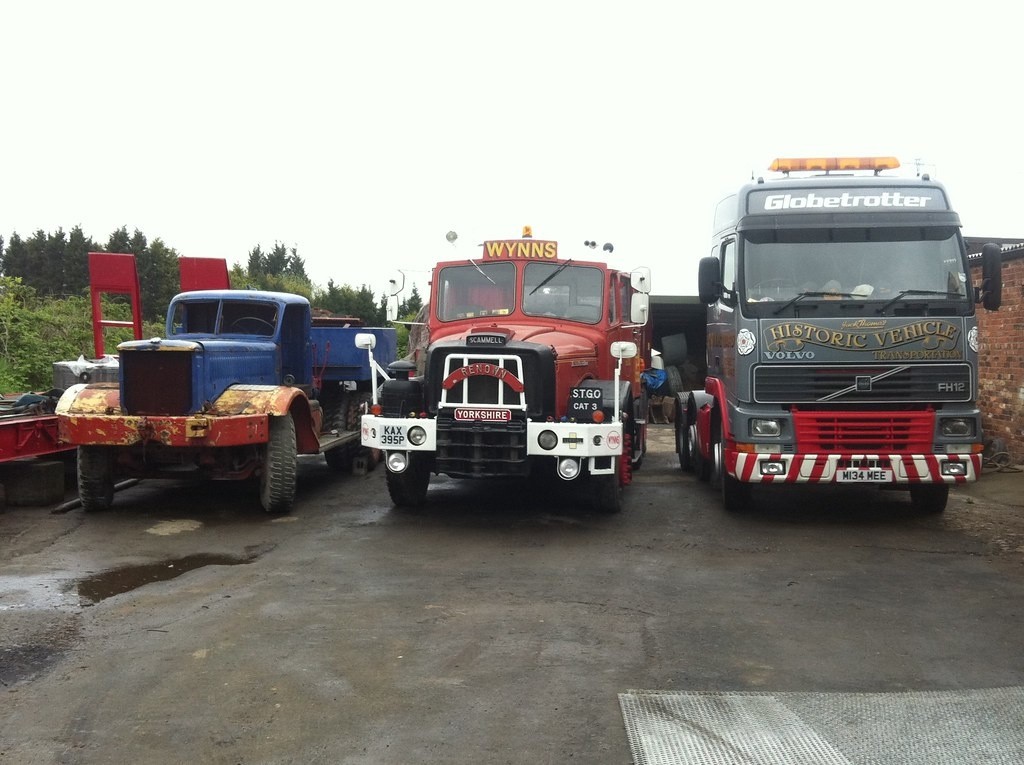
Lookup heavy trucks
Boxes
[55,290,398,514]
[354,225,666,516]
[673,157,1003,515]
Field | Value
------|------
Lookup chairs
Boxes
[566,305,599,322]
